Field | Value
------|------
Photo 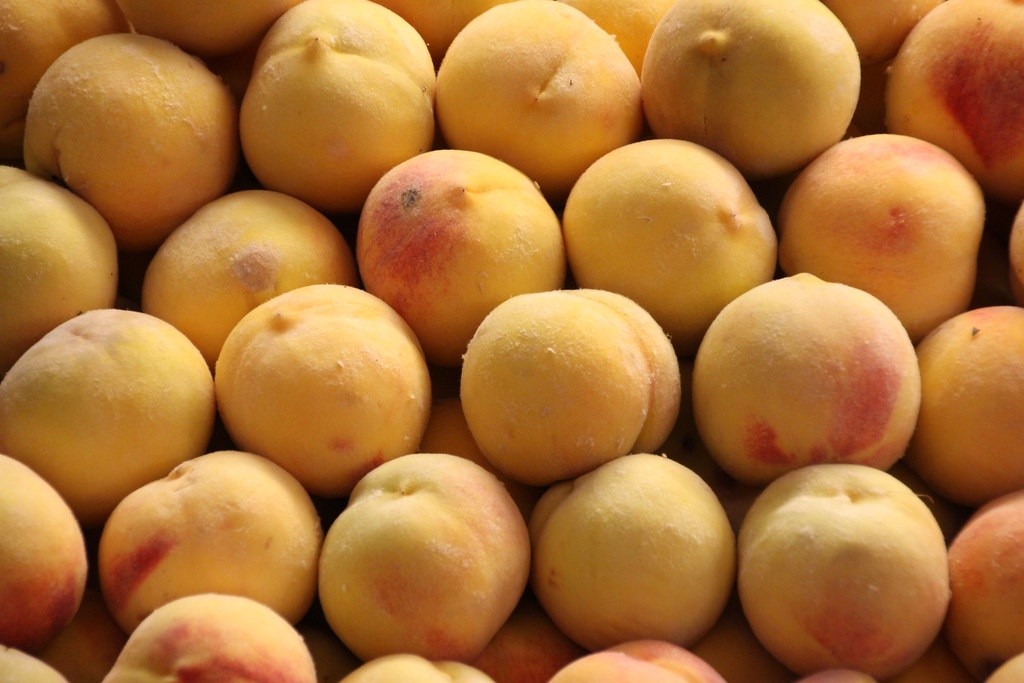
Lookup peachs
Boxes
[0,0,1024,683]
